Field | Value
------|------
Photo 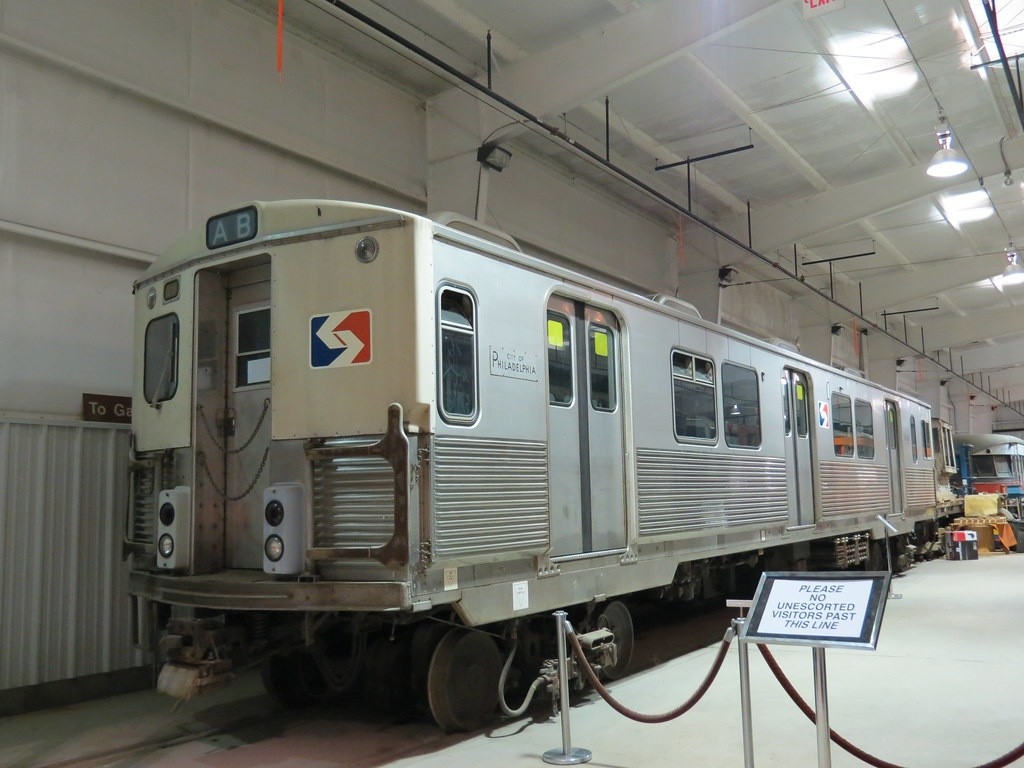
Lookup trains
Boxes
[953,432,1024,518]
[122,197,966,736]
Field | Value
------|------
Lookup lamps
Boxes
[941,380,948,386]
[477,145,511,171]
[719,267,738,283]
[832,325,846,335]
[970,396,977,400]
[896,359,906,367]
